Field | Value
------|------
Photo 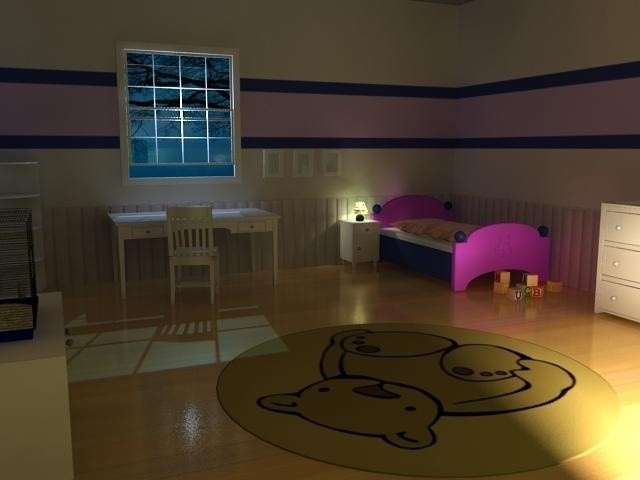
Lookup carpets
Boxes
[215,321,621,478]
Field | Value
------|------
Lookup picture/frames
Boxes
[321,149,344,175]
[262,148,285,179]
[291,149,315,179]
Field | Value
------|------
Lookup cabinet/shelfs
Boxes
[0,160,48,295]
[593,202,640,323]
[0,291,76,480]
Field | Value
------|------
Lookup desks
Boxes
[107,208,283,300]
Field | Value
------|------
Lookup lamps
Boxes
[352,201,369,220]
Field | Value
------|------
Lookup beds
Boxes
[370,194,552,292]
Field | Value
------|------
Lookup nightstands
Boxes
[337,219,383,271]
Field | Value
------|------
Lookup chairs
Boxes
[166,206,220,305]
